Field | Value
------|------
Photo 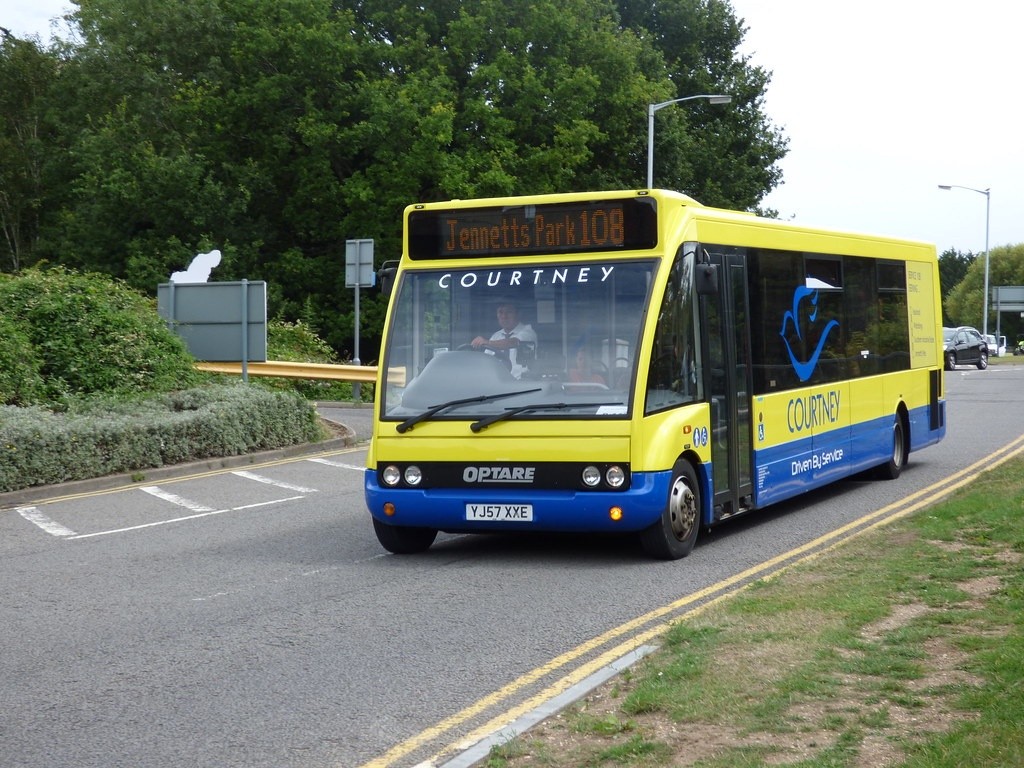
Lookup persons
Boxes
[470,294,537,380]
[570,346,604,393]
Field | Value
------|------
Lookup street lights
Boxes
[937,184,990,341]
[647,94,733,190]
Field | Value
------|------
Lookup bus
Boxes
[364,188,948,561]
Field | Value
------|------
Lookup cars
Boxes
[979,334,1006,358]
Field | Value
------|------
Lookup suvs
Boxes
[942,326,989,372]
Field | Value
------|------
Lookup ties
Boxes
[504,332,513,358]
